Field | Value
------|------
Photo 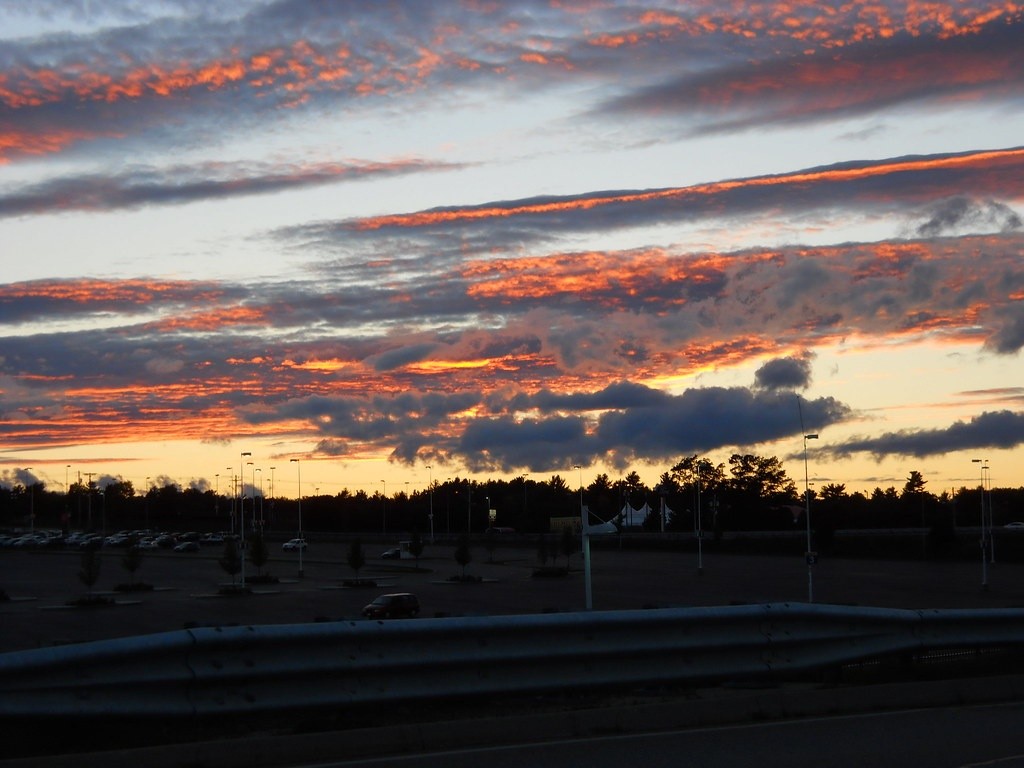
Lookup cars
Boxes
[0,528,240,552]
[283,539,308,550]
[1004,522,1024,529]
[380,548,400,559]
[361,593,420,619]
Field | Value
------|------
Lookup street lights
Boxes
[227,467,233,534]
[523,474,529,504]
[240,453,251,588]
[982,467,996,563]
[246,462,255,521]
[98,493,105,530]
[291,459,304,578]
[316,487,319,496]
[447,489,458,533]
[698,464,706,569]
[574,466,583,507]
[972,460,989,584]
[804,434,818,602]
[381,480,385,533]
[486,496,490,509]
[426,466,433,544]
[256,469,263,543]
[84,473,96,521]
[215,474,219,515]
[66,465,70,493]
[270,467,275,520]
[865,490,868,498]
